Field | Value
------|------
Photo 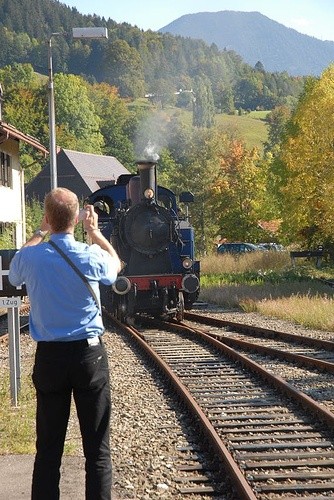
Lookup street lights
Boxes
[46,27,107,191]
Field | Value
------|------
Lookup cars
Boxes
[216,243,261,255]
[256,241,279,252]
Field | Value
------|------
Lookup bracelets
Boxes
[87,228,101,234]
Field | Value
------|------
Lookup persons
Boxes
[8,188,124,500]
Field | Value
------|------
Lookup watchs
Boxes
[33,229,46,238]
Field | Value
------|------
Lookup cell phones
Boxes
[78,208,86,221]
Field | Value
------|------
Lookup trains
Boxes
[81,155,201,327]
